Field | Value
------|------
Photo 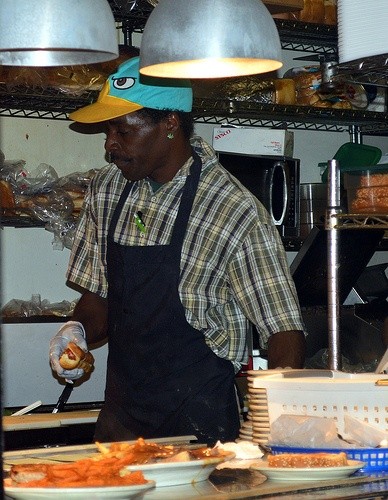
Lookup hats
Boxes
[68,56,193,123]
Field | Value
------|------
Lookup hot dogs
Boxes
[58,342,94,373]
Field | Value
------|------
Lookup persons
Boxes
[49,57,308,441]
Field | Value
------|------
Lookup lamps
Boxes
[138,0,283,79]
[0,0,120,67]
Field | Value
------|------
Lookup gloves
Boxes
[49,321,95,380]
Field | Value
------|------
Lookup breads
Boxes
[0,180,86,218]
[288,0,338,26]
[267,452,347,469]
[271,66,353,111]
[0,56,130,92]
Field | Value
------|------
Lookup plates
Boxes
[250,453,365,482]
[3,473,156,500]
[124,451,235,487]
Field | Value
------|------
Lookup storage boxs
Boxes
[213,125,294,156]
[253,370,388,430]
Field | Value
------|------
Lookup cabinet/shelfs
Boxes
[0,17,388,324]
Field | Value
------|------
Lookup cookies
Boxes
[239,369,295,443]
